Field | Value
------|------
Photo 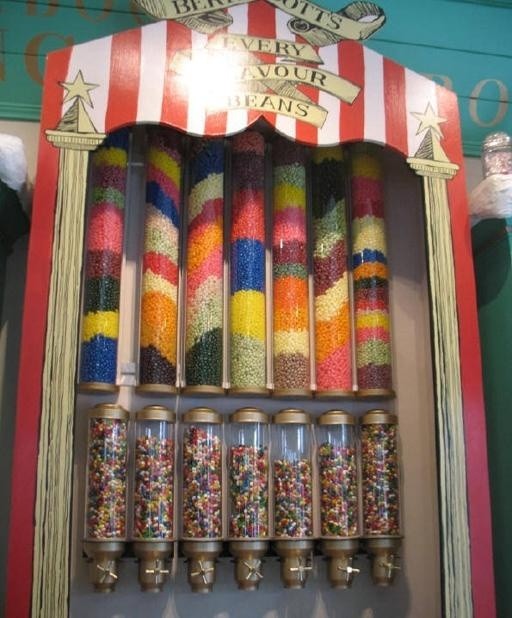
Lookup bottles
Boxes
[482,131,511,178]
[132,402,175,593]
[227,407,271,593]
[322,410,359,590]
[275,408,316,592]
[175,403,227,591]
[362,407,402,587]
[86,403,127,593]
